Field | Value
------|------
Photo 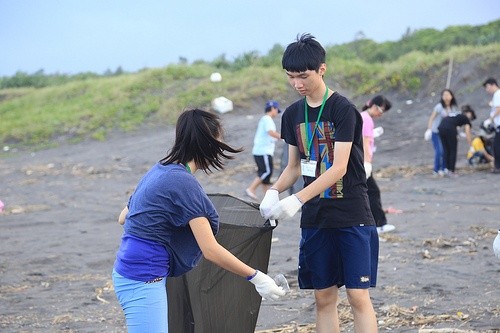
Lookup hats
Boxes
[265,101,283,113]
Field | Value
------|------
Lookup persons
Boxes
[246,100,282,200]
[466,134,495,166]
[260,32,380,333]
[424,88,457,176]
[112,107,287,333]
[439,105,476,173]
[359,95,395,233]
[483,79,500,174]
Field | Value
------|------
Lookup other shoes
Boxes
[432,170,445,178]
[445,169,457,177]
[376,224,395,233]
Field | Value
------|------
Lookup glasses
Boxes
[375,105,385,114]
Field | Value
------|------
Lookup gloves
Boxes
[247,270,285,302]
[260,189,303,221]
[493,231,500,259]
[374,127,384,137]
[364,162,372,180]
[425,129,432,141]
